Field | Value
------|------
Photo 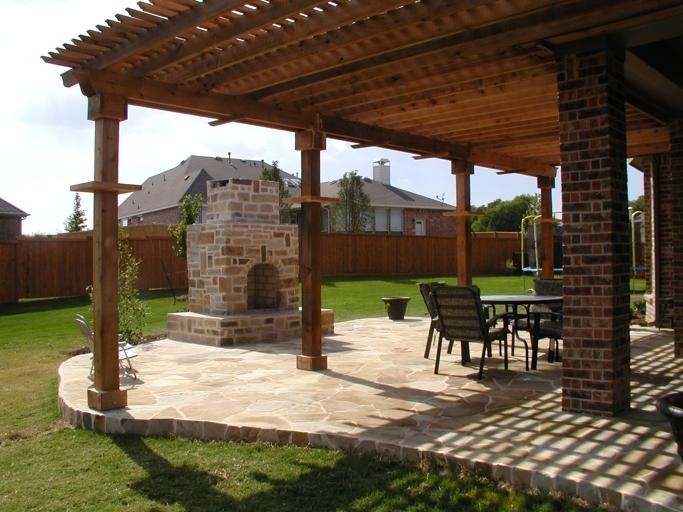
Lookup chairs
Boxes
[415,277,562,379]
[74,314,139,382]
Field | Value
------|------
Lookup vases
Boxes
[381,296,411,320]
